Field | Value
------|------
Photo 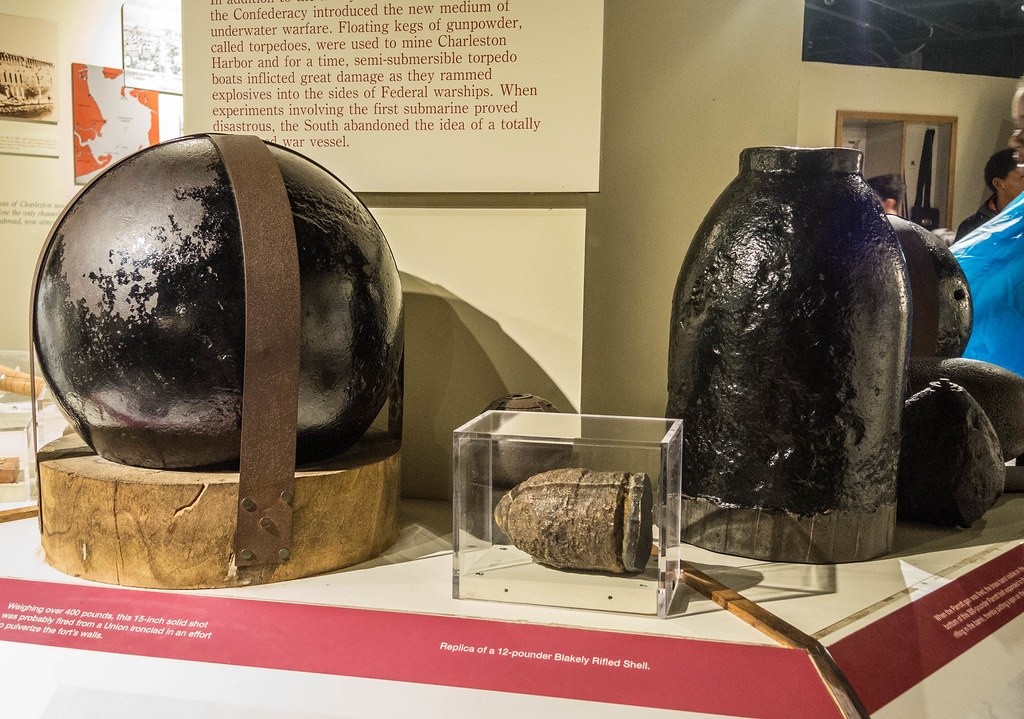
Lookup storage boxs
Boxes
[451,409,685,617]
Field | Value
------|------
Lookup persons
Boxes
[867,173,906,216]
[930,73,1024,379]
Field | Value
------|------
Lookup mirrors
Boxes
[835,109,959,231]
